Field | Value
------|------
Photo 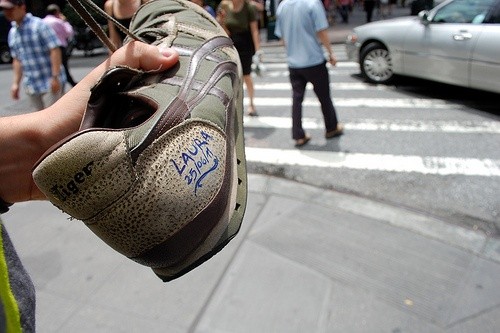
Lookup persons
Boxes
[274,0,345,146]
[0,38,180,333]
[0,0,438,118]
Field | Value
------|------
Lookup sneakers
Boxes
[31,0,248,283]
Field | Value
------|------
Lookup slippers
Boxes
[325,123,343,137]
[294,133,311,147]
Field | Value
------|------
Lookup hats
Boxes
[0,0,22,8]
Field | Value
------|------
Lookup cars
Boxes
[0,11,14,64]
[344,0,500,93]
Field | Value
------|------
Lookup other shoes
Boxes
[248,111,259,116]
